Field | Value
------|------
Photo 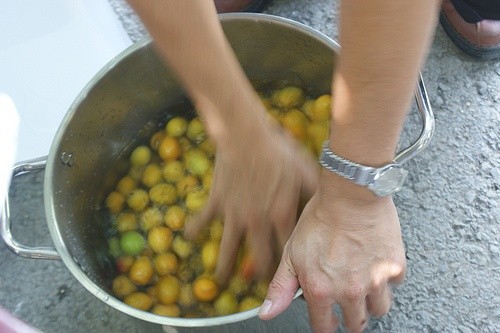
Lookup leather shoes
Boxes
[441,0,500,61]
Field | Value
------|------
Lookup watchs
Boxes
[318,139,409,196]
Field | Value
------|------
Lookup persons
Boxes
[127,0,500,331]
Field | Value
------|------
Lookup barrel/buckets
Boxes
[1,12,436,332]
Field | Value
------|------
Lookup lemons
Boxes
[105,87,332,319]
